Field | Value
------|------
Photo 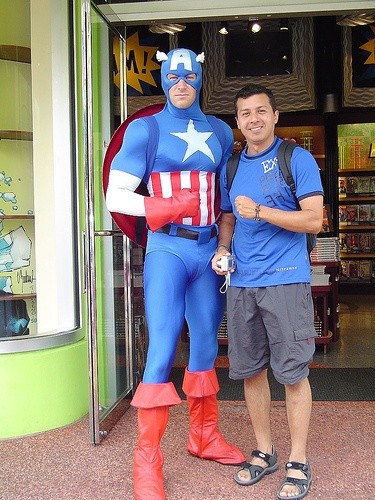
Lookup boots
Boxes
[130,381,182,500]
[182,367,247,465]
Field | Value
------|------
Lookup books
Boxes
[115,232,144,339]
[309,175,375,337]
[187,310,229,339]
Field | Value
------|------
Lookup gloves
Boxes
[283,137,296,142]
[145,188,198,233]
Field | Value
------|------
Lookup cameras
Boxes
[216,255,236,272]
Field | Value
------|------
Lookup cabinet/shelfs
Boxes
[113,106,375,357]
[0,45,37,301]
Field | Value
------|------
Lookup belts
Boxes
[160,224,218,240]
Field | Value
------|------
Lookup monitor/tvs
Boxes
[225,28,293,76]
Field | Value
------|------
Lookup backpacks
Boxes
[226,140,316,265]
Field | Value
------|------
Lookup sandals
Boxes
[234,444,279,486]
[277,460,312,500]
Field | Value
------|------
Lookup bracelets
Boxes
[216,244,231,253]
[254,203,261,224]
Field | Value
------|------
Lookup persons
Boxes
[211,84,324,500]
[106,46,246,500]
